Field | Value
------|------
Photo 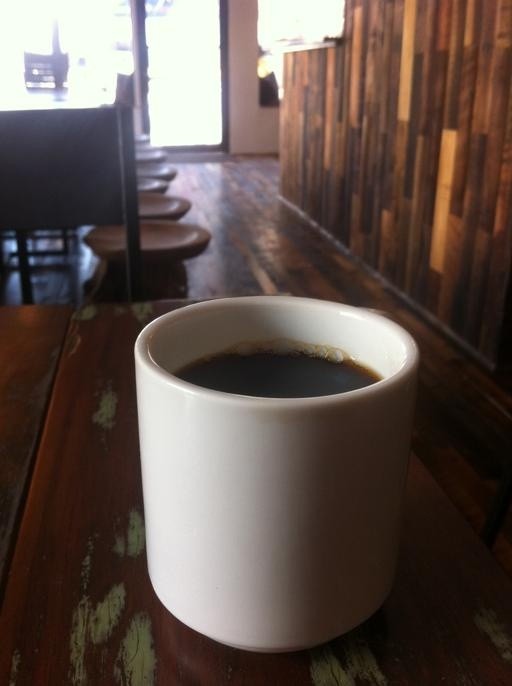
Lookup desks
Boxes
[0,294,512,686]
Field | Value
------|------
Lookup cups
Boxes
[130,295,424,651]
